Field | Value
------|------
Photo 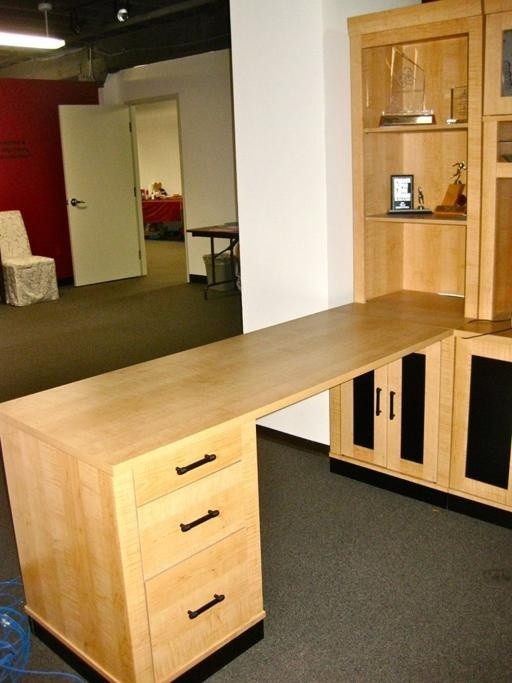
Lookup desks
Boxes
[0,303,454,683]
[186,225,240,300]
[141,199,183,239]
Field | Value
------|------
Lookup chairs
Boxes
[0,209,60,307]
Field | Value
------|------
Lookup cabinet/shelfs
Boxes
[477,13,511,330]
[340,336,439,483]
[453,337,512,508]
[363,16,484,317]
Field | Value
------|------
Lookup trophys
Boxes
[378,45,437,126]
[433,159,467,218]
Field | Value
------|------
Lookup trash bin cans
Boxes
[203,252,237,291]
